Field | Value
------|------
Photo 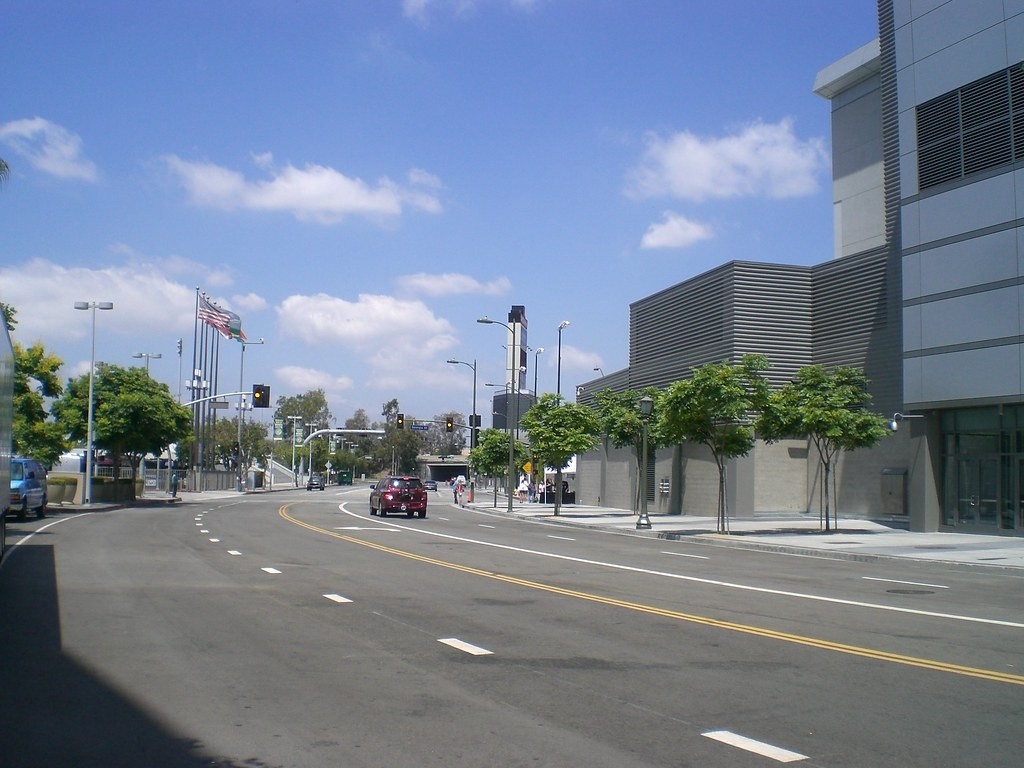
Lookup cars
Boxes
[424,481,438,491]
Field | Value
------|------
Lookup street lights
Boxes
[485,383,539,494]
[74,300,113,505]
[554,321,570,516]
[288,415,318,488]
[477,317,515,513]
[447,358,476,503]
[516,347,545,491]
[176,338,264,491]
[636,392,654,530]
[133,352,162,497]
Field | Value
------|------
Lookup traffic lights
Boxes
[252,384,263,405]
[397,414,404,430]
[447,417,453,431]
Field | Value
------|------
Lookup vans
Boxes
[6,459,48,517]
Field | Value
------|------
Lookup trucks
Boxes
[0,307,17,565]
[338,470,352,484]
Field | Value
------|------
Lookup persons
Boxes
[172,472,178,498]
[453,477,463,504]
[518,478,544,503]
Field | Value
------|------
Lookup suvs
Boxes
[450,474,467,487]
[369,475,427,518]
[307,477,324,491]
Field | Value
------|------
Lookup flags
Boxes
[196,293,248,340]
